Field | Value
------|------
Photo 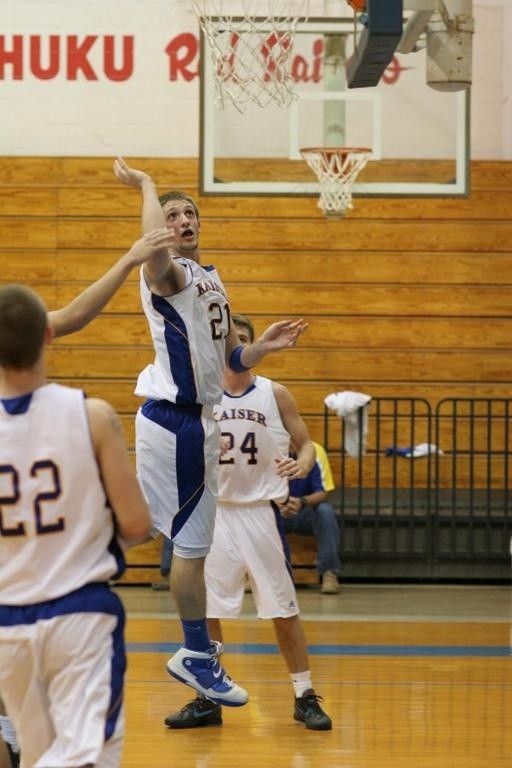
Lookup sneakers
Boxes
[165,638,250,708]
[293,688,333,731]
[321,571,340,595]
[164,697,222,729]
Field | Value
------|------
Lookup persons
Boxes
[0,228,176,768]
[0,282,153,768]
[274,436,343,594]
[114,156,309,707]
[163,312,334,730]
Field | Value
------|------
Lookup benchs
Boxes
[0,157,511,588]
